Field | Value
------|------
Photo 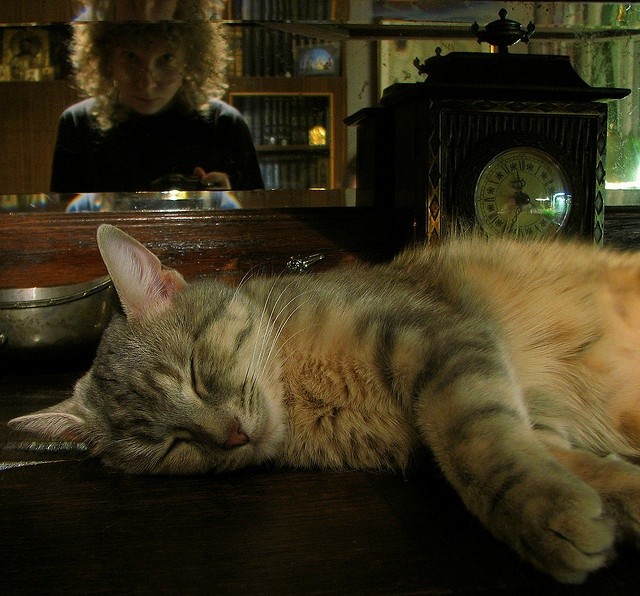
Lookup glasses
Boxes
[106,55,186,84]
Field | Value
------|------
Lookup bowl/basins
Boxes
[2,276,111,348]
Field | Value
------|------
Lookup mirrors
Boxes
[0,0,640,220]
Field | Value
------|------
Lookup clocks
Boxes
[473,147,572,242]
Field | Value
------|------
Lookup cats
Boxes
[6,219,640,584]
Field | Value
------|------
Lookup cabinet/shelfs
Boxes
[220,76,348,192]
[222,0,348,75]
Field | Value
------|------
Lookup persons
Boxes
[73,1,227,24]
[49,22,260,194]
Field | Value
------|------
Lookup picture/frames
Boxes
[370,16,499,106]
